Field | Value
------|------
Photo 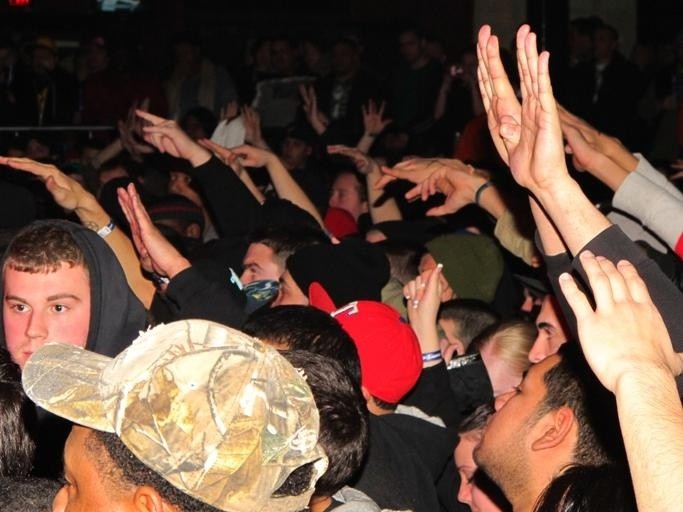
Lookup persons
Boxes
[2,1,682,512]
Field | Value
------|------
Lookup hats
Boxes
[514,274,557,296]
[147,194,207,225]
[21,318,329,512]
[309,283,422,404]
[427,230,506,304]
